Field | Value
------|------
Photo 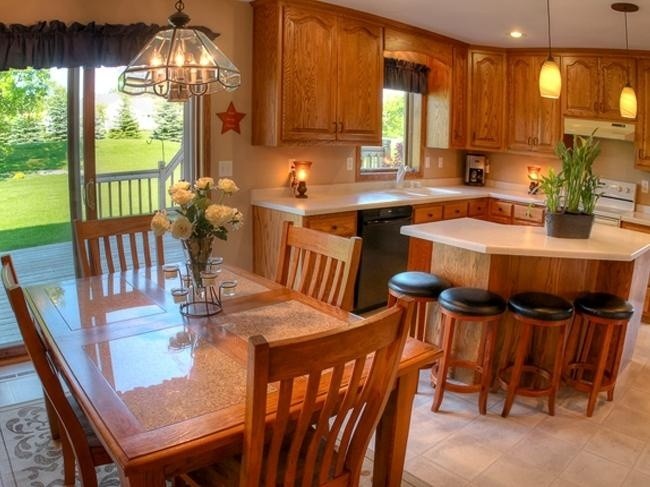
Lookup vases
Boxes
[179,234,224,318]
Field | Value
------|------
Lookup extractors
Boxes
[565,118,636,147]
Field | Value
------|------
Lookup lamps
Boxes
[117,1,241,103]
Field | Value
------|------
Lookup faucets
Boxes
[396,165,409,186]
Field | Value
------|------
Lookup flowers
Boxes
[151,176,245,242]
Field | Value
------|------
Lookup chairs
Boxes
[0,252,116,487]
[71,210,165,277]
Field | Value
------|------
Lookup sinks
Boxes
[397,186,460,198]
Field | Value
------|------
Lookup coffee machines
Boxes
[464,153,486,186]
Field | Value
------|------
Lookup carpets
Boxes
[0,390,434,487]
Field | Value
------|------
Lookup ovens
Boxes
[355,204,413,314]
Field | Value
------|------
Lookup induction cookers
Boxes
[554,197,635,213]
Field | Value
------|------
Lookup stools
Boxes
[385,270,634,417]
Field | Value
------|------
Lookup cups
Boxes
[208,257,223,273]
[162,263,179,278]
[223,279,237,296]
[199,270,217,286]
[171,286,187,304]
